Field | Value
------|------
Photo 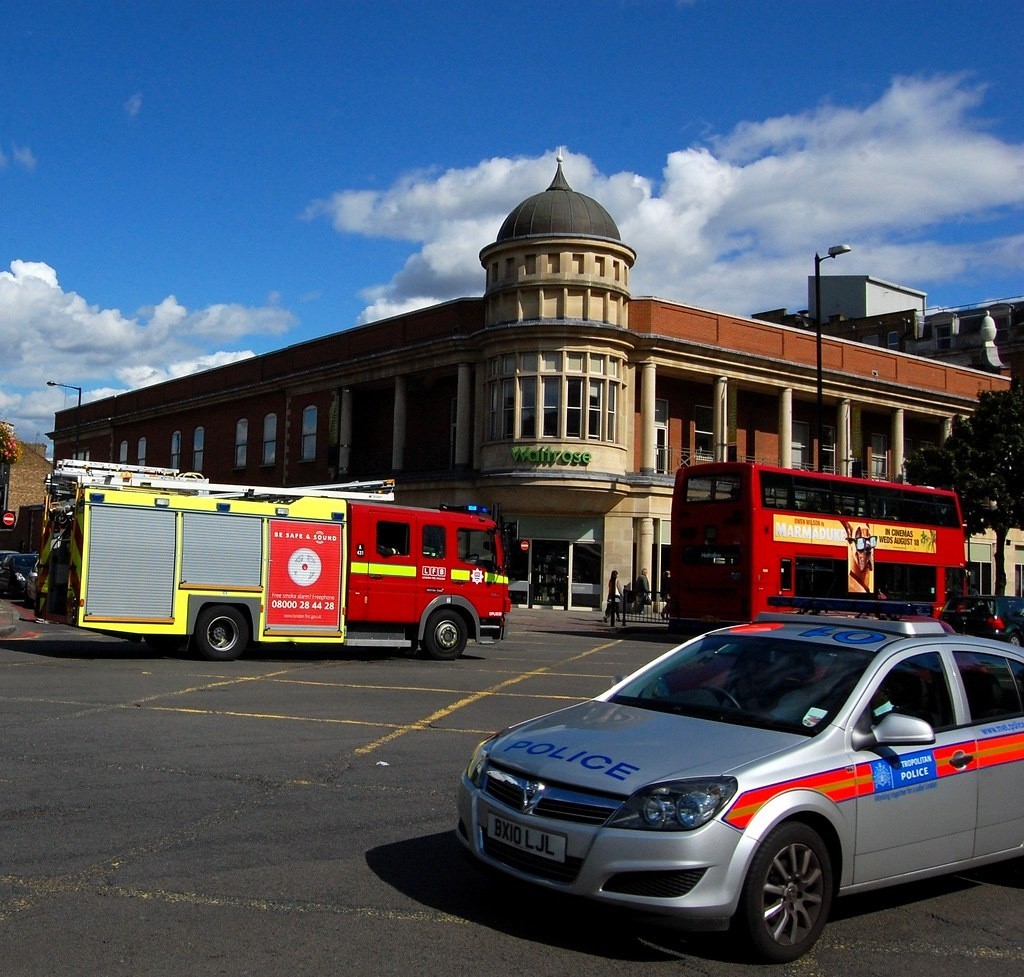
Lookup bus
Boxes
[669,459,972,632]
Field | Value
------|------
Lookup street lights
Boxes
[814,243,852,471]
[47,381,82,459]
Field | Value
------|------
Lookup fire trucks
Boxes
[40,459,520,662]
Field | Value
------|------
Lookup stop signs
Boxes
[521,541,529,551]
[3,513,14,525]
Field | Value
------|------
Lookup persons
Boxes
[660,570,670,620]
[603,570,623,623]
[878,589,887,599]
[969,584,979,595]
[32,557,50,624]
[377,531,400,556]
[635,568,650,615]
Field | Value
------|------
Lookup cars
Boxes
[24,556,40,609]
[453,595,1024,965]
[0,550,20,571]
[939,592,1024,647]
[0,552,39,599]
